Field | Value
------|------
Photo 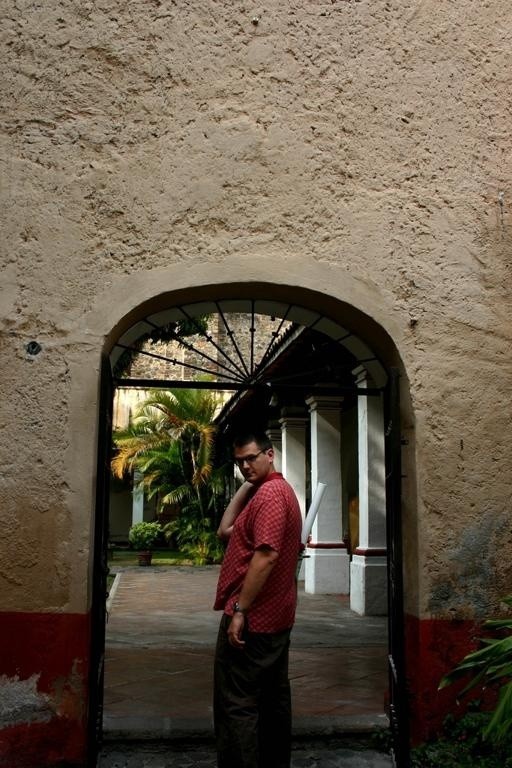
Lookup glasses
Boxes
[233,447,268,469]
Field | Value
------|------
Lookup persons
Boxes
[210,432,303,768]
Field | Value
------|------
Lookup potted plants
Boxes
[128,519,163,566]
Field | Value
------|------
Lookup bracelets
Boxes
[232,601,247,616]
